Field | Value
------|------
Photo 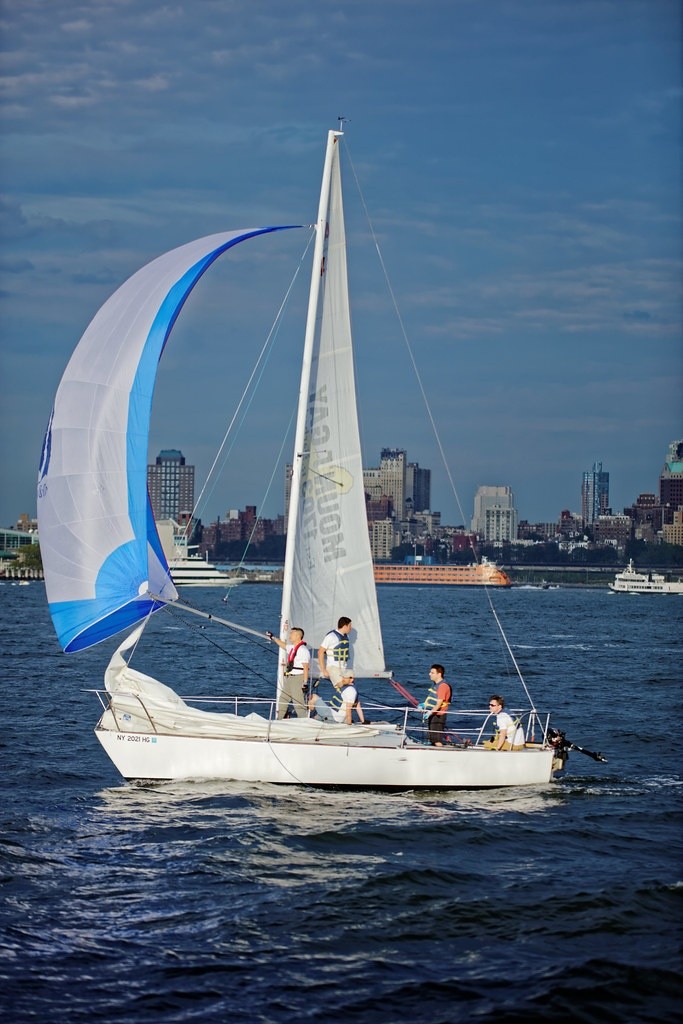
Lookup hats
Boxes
[339,671,355,678]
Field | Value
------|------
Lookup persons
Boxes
[265,627,310,720]
[416,664,453,747]
[482,695,525,751]
[307,669,372,725]
[317,617,352,690]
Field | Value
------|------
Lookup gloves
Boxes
[302,683,308,693]
[362,720,370,724]
[418,703,424,708]
[266,631,273,638]
[424,713,431,719]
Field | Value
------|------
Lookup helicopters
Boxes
[34,129,567,785]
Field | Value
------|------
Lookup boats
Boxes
[373,562,511,588]
[155,518,245,587]
[607,558,683,595]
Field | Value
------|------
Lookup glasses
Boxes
[489,704,496,707]
